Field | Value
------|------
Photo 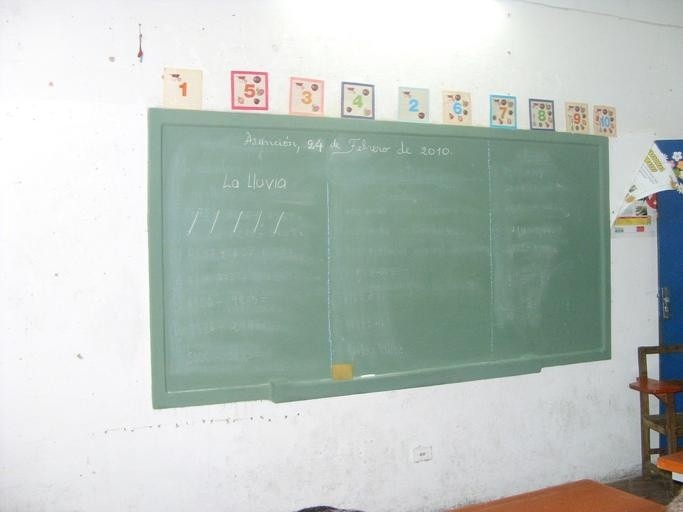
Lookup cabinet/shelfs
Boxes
[630,344,683,480]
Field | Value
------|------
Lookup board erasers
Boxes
[333,362,353,382]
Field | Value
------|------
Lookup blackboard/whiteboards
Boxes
[147,108,612,409]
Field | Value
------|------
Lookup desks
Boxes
[656,450,683,483]
[452,479,669,512]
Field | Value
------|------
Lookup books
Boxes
[657,451,682,482]
